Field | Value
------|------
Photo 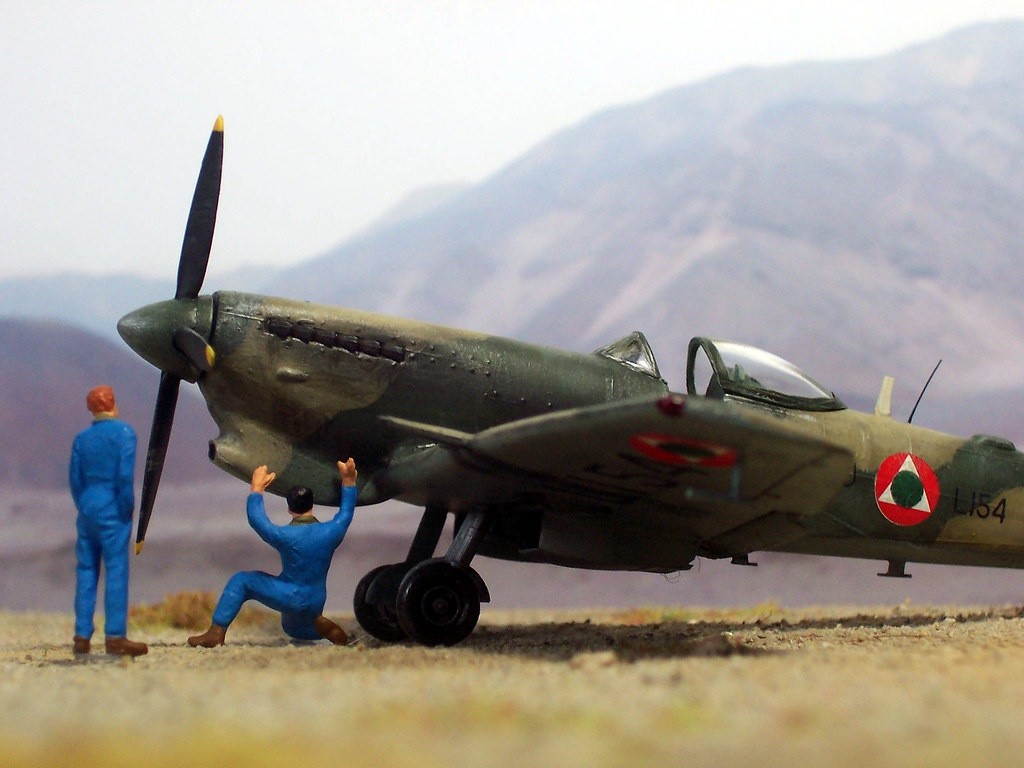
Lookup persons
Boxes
[187,458,358,647]
[69,387,147,657]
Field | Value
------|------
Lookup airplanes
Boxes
[114,114,1024,651]
